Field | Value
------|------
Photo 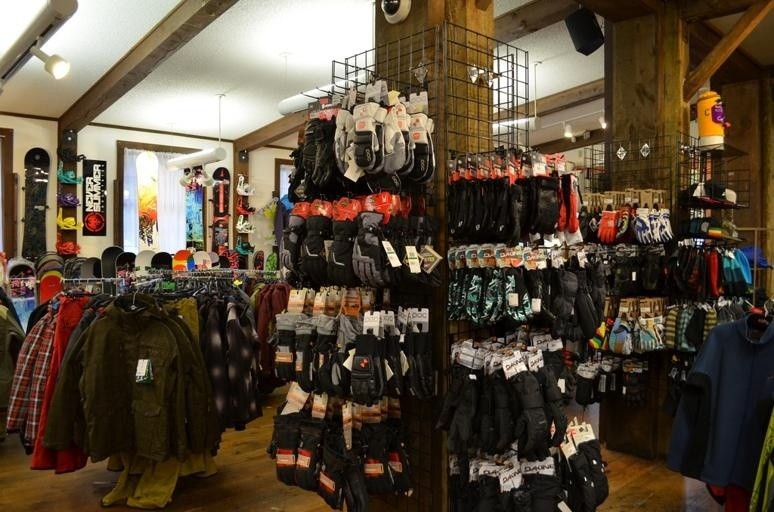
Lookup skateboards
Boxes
[185,165,205,250]
[22,148,50,262]
[208,167,230,255]
[101,246,276,294]
[137,151,158,252]
[0,251,100,334]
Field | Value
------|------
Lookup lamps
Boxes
[30,46,70,79]
[598,116,606,130]
[561,122,572,138]
[493,62,540,136]
[278,52,376,114]
[167,93,227,186]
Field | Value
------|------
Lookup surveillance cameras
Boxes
[381,0,411,25]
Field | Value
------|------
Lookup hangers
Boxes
[174,268,288,298]
[751,298,773,334]
[60,277,164,313]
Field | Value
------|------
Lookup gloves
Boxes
[447,170,595,343]
[272,313,433,406]
[436,349,610,512]
[267,406,418,512]
[585,206,675,407]
[279,101,438,288]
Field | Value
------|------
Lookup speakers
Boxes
[565,7,604,56]
